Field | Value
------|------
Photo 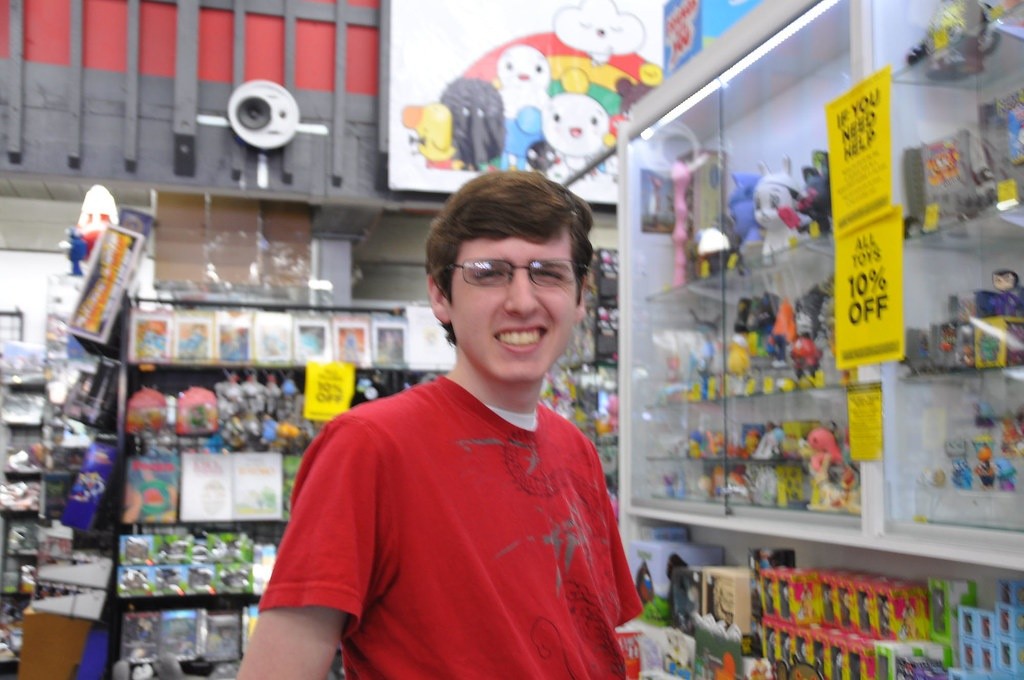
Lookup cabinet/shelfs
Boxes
[614,0,1024,680]
[111,291,469,680]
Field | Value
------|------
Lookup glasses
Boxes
[445,258,582,287]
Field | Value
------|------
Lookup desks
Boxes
[21,605,97,680]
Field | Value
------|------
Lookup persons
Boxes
[233,171,644,680]
[67,225,86,275]
[991,270,1022,315]
